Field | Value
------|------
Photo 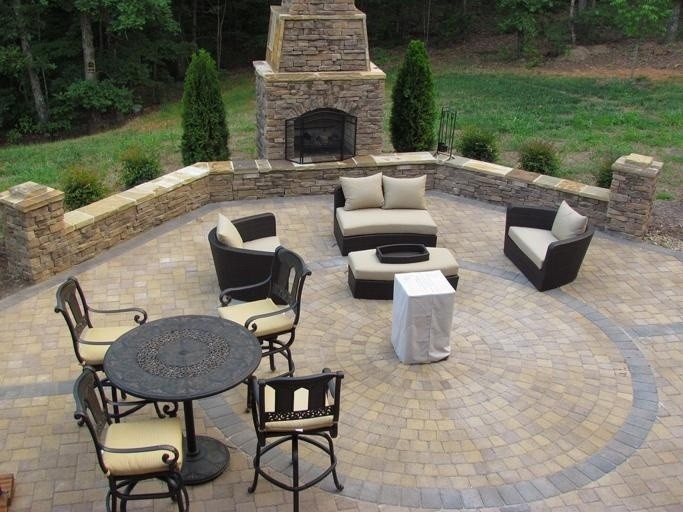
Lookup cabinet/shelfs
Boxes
[391,270,456,364]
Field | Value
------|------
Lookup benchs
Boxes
[348,248,459,299]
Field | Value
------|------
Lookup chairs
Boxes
[208,212,289,306]
[216,246,312,383]
[504,202,595,292]
[245,367,345,512]
[73,366,190,512]
[54,276,148,426]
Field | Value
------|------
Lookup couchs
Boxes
[333,182,437,257]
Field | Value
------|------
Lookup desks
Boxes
[103,315,261,486]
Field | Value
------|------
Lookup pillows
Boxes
[215,213,244,249]
[550,200,589,242]
[339,172,385,210]
[382,174,428,210]
